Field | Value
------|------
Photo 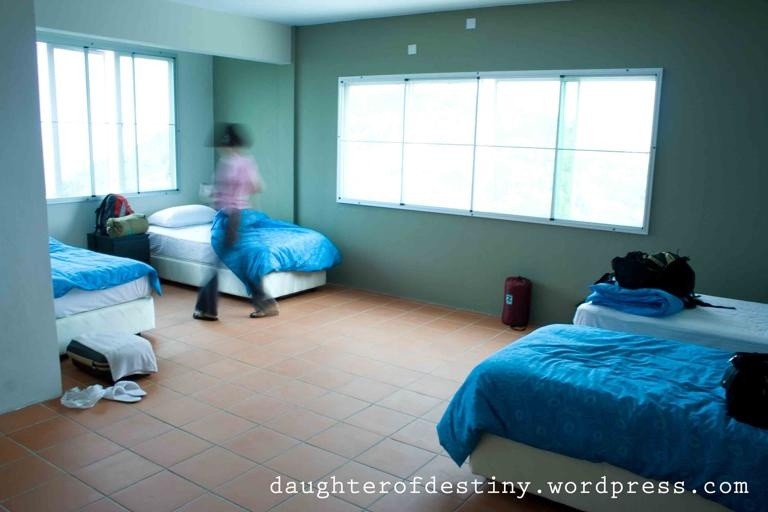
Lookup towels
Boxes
[73,331,159,382]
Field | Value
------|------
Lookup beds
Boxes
[48,235,159,357]
[425,318,766,512]
[570,290,767,357]
[150,201,332,305]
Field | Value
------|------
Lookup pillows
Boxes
[147,200,221,231]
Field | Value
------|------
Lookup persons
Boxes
[192,120,280,321]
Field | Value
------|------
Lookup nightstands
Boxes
[85,229,152,267]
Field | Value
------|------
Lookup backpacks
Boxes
[95,194,134,236]
[593,251,736,310]
[718,351,767,430]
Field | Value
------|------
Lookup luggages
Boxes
[65,333,150,382]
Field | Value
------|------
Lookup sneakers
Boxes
[250,308,279,318]
[192,311,217,321]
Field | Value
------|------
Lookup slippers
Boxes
[101,386,141,403]
[113,381,146,397]
